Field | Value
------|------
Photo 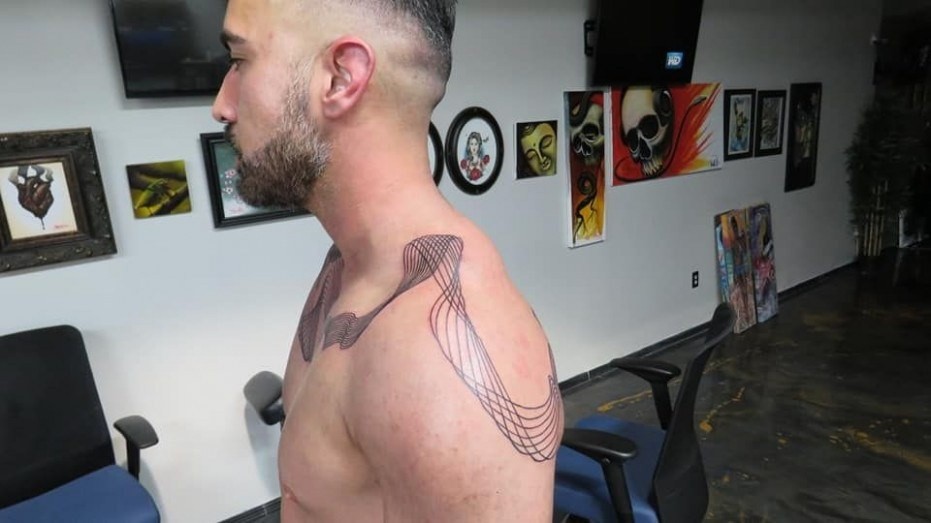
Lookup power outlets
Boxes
[692,271,699,288]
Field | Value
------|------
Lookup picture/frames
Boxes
[426,121,445,185]
[199,131,313,230]
[755,89,786,158]
[1,126,118,276]
[784,80,822,193]
[721,87,754,161]
[444,105,505,196]
[107,1,231,100]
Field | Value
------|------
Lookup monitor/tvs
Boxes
[589,1,704,85]
[110,0,228,100]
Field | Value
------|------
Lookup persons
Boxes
[213,0,563,523]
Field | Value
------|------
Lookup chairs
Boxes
[1,324,163,523]
[550,301,738,523]
